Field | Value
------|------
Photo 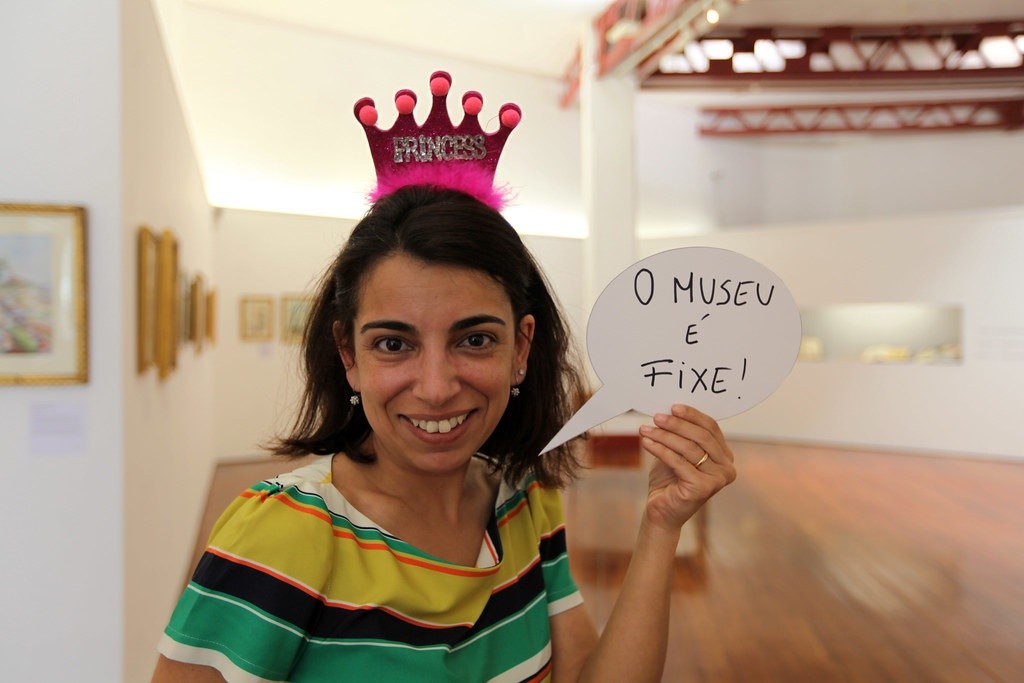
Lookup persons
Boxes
[151,182,737,683]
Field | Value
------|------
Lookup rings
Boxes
[694,453,709,468]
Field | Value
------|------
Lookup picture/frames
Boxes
[0,201,89,388]
[131,226,319,383]
